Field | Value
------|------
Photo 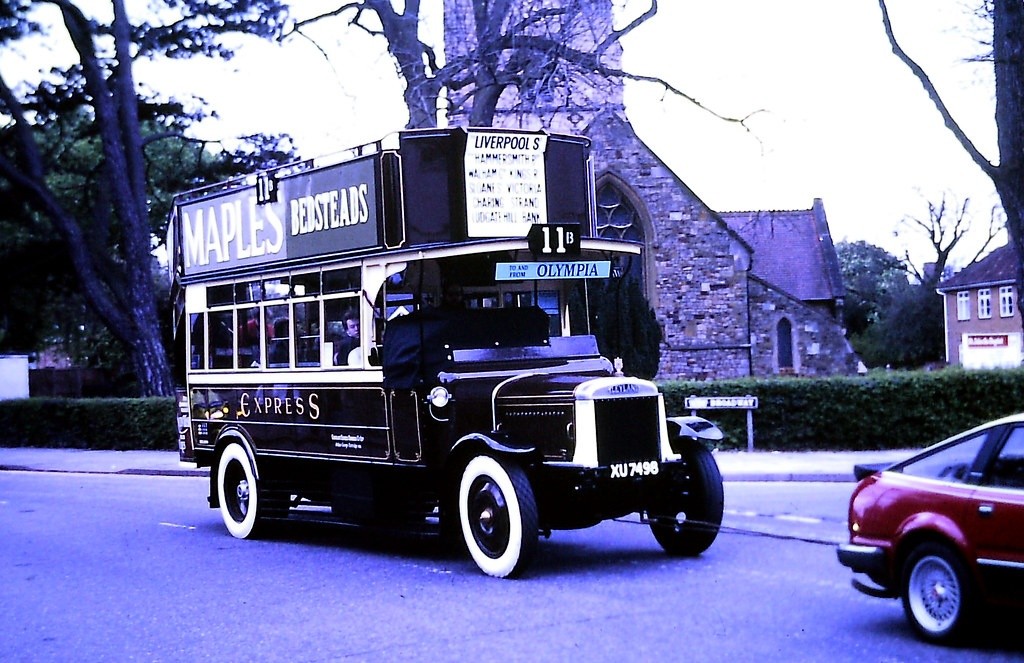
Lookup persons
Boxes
[238,308,320,364]
[337,310,361,366]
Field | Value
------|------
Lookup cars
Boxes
[832,409,1024,646]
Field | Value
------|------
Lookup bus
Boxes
[171,125,727,577]
